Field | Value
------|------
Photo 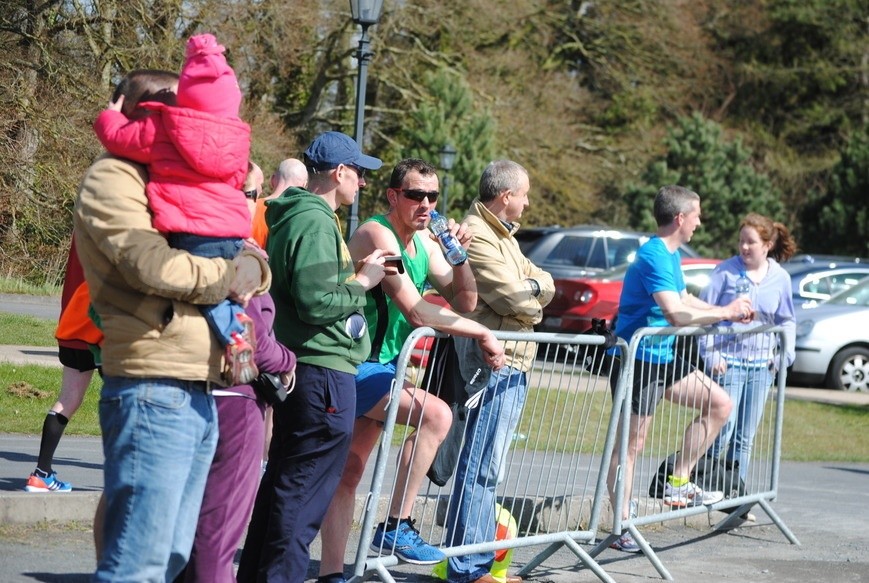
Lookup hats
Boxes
[304,130,384,172]
[177,33,242,120]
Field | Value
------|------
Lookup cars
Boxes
[781,248,869,325]
[794,280,869,392]
[537,257,729,374]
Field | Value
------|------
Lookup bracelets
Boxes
[243,189,257,203]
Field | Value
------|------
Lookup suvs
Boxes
[511,227,696,267]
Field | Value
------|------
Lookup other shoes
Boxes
[746,512,756,521]
[608,530,652,554]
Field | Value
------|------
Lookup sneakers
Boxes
[226,334,259,384]
[371,515,445,565]
[665,481,724,506]
[26,470,72,494]
[238,315,256,349]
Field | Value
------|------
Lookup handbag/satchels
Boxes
[650,450,748,499]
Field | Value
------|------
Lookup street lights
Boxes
[439,141,458,218]
[349,0,386,248]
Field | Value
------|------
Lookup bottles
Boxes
[735,270,751,319]
[428,209,466,265]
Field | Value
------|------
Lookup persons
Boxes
[695,212,801,486]
[238,130,397,583]
[431,157,555,583]
[24,30,310,583]
[317,157,478,583]
[93,33,260,386]
[606,185,756,553]
[72,67,274,583]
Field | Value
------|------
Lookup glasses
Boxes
[395,187,439,204]
[347,163,366,177]
